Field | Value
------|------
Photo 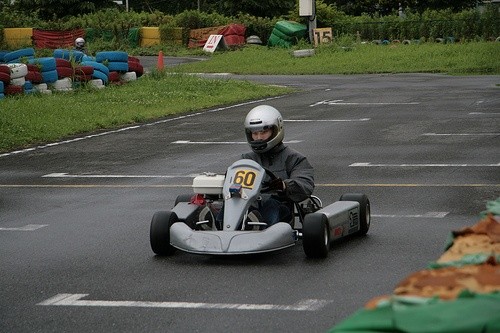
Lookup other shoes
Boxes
[245,210,263,231]
[198,207,218,231]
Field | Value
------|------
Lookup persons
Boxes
[195,105,315,231]
[72,38,88,54]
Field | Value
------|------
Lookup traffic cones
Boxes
[157,51,165,72]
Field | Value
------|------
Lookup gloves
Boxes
[261,177,284,191]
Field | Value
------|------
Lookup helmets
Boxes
[75,37,85,49]
[244,105,284,152]
